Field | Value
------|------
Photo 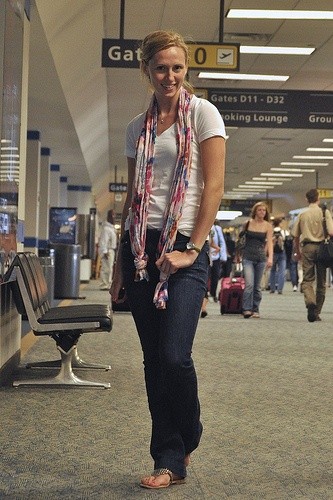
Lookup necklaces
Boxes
[156,102,180,124]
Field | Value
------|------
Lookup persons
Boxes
[108,31,227,490]
[291,188,333,322]
[210,219,228,302]
[99,209,118,289]
[199,229,221,318]
[234,201,275,319]
[224,217,333,294]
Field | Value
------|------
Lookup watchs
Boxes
[184,242,203,255]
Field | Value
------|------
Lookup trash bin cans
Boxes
[38,247,55,309]
[51,243,81,298]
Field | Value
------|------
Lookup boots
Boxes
[201,298,208,318]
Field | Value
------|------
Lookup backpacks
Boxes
[273,231,284,254]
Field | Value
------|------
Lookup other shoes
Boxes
[243,310,261,318]
[307,302,322,322]
[207,283,305,304]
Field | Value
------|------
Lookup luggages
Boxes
[218,260,246,315]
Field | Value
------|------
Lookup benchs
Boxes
[3,249,114,391]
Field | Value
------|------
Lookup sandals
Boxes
[139,468,186,488]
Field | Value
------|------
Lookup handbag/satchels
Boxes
[320,208,333,267]
[236,220,250,250]
